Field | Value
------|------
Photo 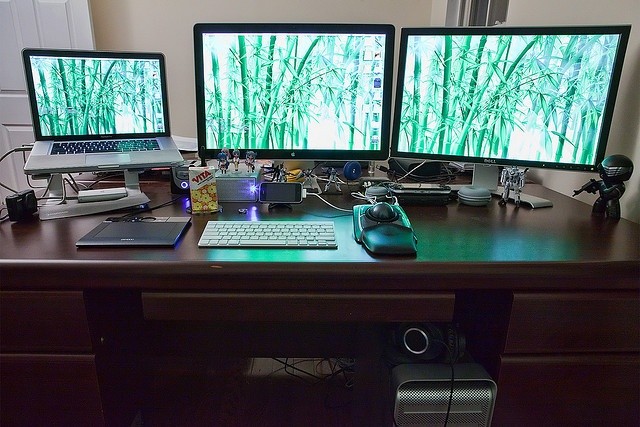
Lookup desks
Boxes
[1,172,639,427]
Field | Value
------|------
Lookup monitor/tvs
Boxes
[193,23,396,194]
[387,23,632,208]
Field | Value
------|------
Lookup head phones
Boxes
[401,322,481,367]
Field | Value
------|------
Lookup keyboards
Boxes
[198,218,338,250]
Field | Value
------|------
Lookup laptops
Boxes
[19,46,184,175]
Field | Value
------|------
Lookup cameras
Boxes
[5,188,40,224]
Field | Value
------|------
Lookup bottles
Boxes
[188,165,218,211]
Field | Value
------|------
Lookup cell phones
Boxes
[258,181,304,203]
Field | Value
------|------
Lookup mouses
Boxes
[353,203,419,256]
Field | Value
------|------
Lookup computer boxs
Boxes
[376,340,498,427]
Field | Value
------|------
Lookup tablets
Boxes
[74,216,193,250]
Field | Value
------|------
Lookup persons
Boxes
[296,162,323,195]
[571,152,634,220]
[245,150,257,173]
[497,164,530,208]
[232,148,241,172]
[322,164,343,192]
[218,147,231,172]
